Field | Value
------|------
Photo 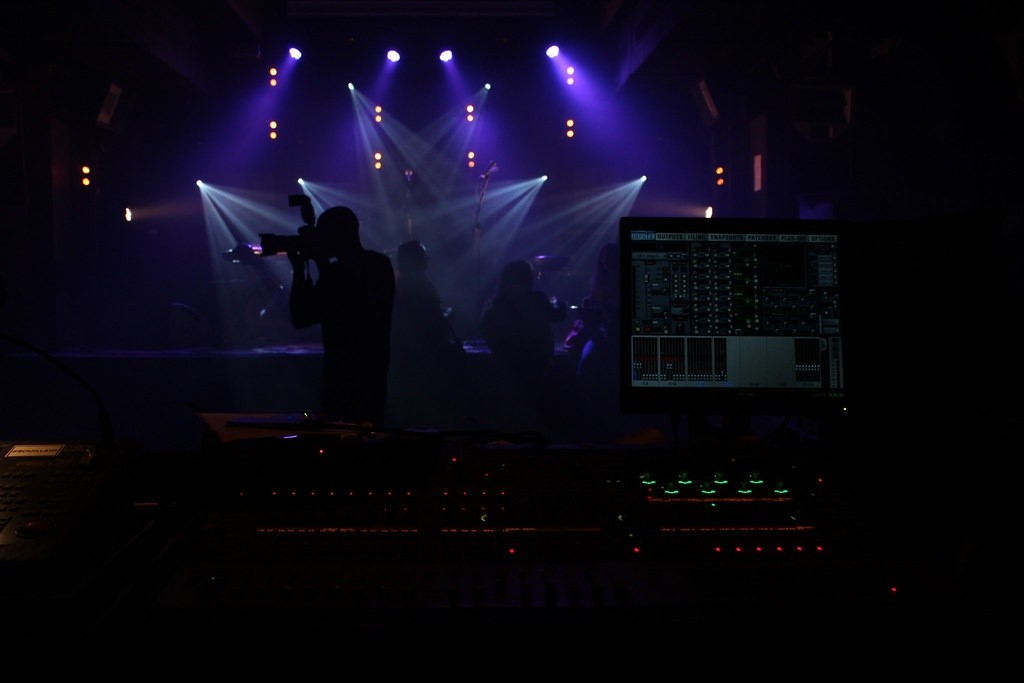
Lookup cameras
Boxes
[259,194,328,258]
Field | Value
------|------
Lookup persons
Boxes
[380,240,468,427]
[574,243,621,343]
[479,257,559,415]
[284,203,397,432]
[572,297,634,442]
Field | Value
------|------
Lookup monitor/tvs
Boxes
[618,215,856,414]
